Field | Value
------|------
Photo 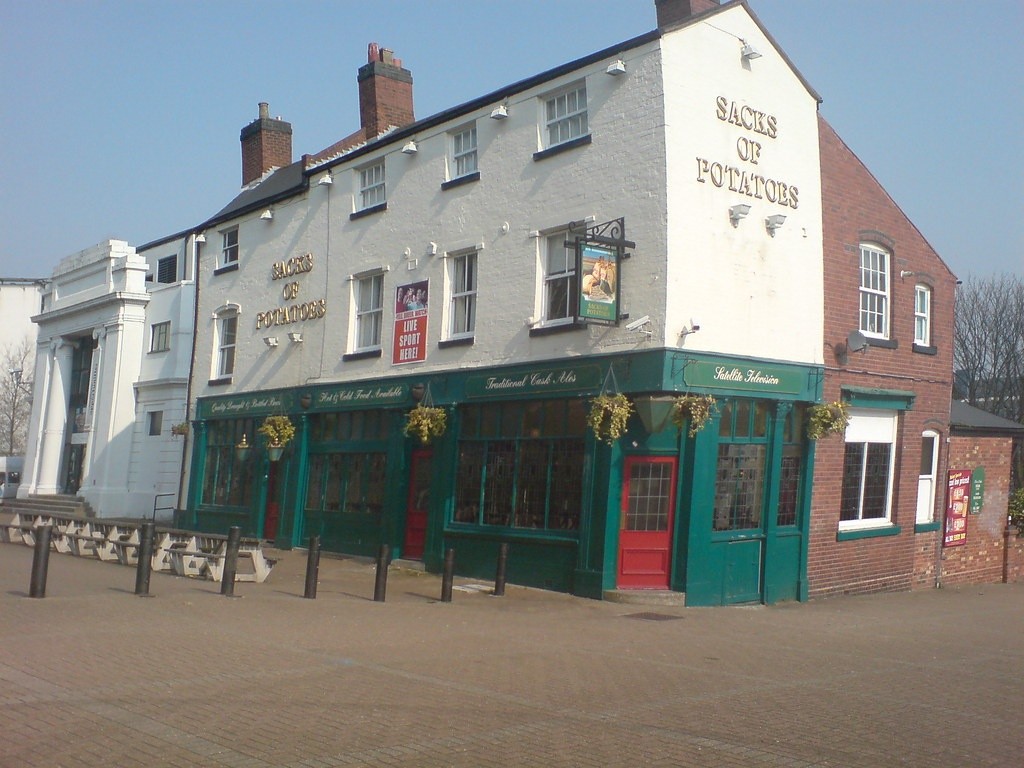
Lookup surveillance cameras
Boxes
[689,317,700,330]
[626,315,650,331]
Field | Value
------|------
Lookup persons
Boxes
[396,287,427,313]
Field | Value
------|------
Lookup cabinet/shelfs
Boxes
[718,455,764,521]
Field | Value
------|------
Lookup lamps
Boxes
[260,209,274,220]
[490,105,509,119]
[606,60,626,76]
[287,333,303,342]
[402,141,418,153]
[730,203,751,229]
[262,337,278,346]
[319,175,334,184]
[741,44,764,59]
[195,234,207,243]
[767,214,787,238]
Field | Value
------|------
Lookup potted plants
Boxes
[171,422,190,443]
[260,415,297,461]
[802,400,853,441]
[671,394,722,439]
[402,402,449,445]
[580,392,637,447]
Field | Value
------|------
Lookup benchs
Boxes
[110,539,163,571]
[63,532,110,563]
[2,524,33,546]
[26,527,65,553]
[263,554,278,573]
[164,548,226,582]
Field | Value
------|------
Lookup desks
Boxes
[0,506,267,583]
